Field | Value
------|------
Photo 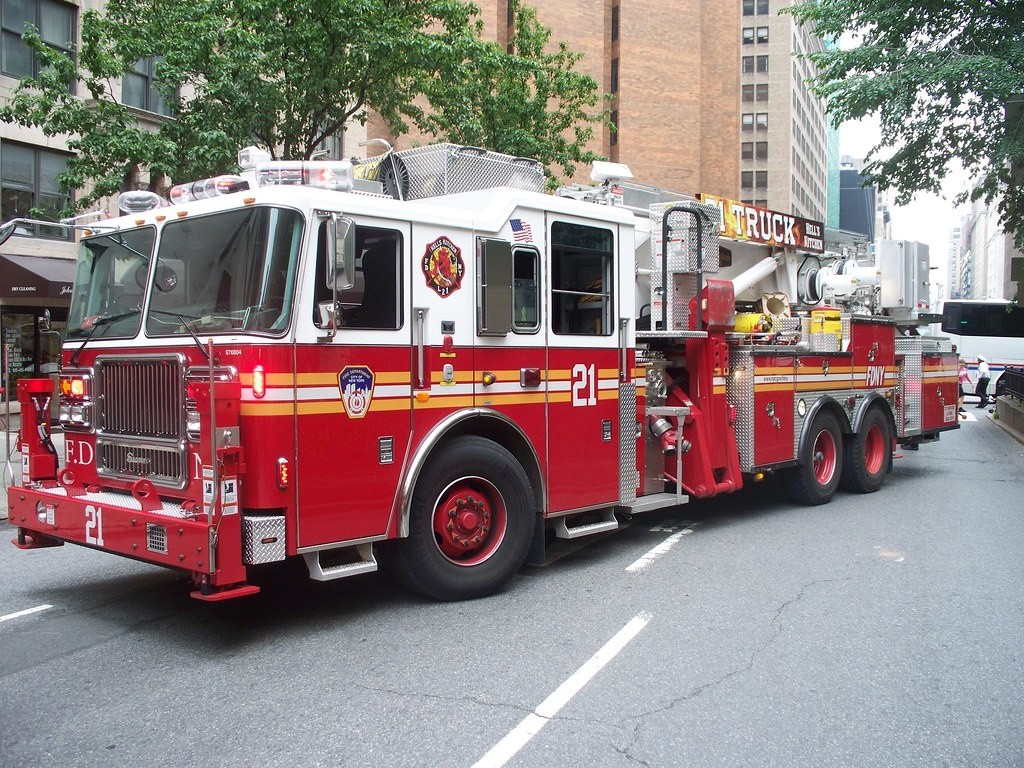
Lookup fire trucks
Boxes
[1,139,961,604]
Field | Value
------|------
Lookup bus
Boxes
[935,299,1024,398]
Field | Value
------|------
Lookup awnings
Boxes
[0,255,125,298]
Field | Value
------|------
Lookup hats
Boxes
[977,355,986,362]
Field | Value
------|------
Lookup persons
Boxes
[958,358,974,412]
[975,355,990,408]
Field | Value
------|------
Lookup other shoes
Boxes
[959,407,966,412]
[975,404,982,408]
[982,400,988,407]
